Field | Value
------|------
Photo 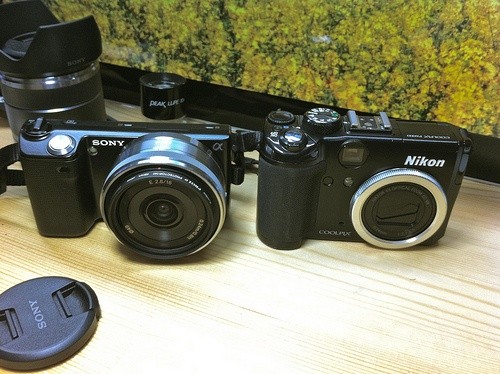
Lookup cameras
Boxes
[254,106,472,254]
[17,114,246,261]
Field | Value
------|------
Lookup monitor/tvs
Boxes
[37,1,499,189]
